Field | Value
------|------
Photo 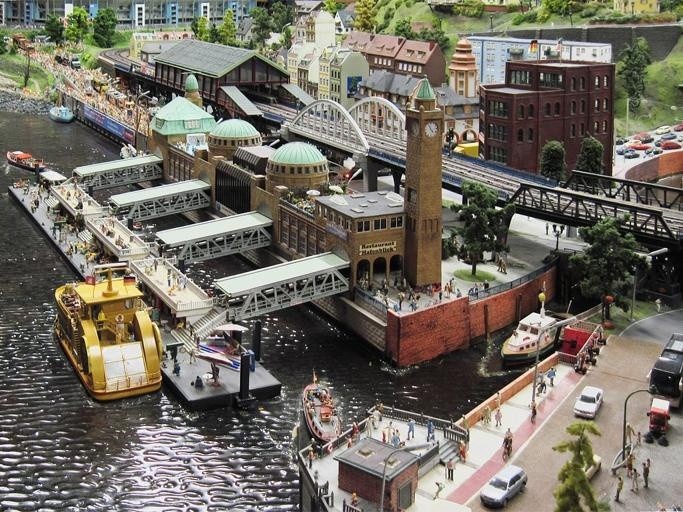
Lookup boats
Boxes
[6,150,46,172]
[38,169,66,187]
[302,369,342,446]
[119,144,132,159]
[52,260,165,403]
[500,292,582,365]
[49,105,74,123]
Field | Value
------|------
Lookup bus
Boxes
[646,332,682,408]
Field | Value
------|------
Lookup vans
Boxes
[647,398,671,435]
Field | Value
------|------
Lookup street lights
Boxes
[378,439,431,512]
[622,389,654,463]
[345,167,363,184]
[133,89,151,147]
[552,224,564,250]
[529,318,577,412]
[628,247,668,323]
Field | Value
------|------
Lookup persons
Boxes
[305,404,467,512]
[356,248,508,310]
[477,365,557,458]
[654,295,662,313]
[14,177,222,392]
[614,422,651,503]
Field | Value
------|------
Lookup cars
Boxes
[3,33,135,111]
[575,386,603,418]
[615,121,683,159]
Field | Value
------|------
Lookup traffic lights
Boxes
[448,128,453,140]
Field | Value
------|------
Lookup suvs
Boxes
[479,464,527,509]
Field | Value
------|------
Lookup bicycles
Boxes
[501,441,514,461]
